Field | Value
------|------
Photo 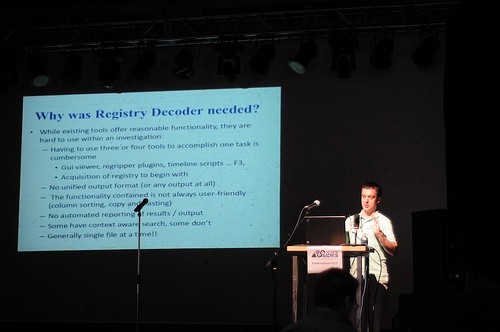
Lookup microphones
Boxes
[134,198,148,212]
[354,215,360,229]
[304,200,320,208]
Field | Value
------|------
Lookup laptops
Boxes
[305,216,346,246]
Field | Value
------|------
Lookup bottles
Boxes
[361,234,369,246]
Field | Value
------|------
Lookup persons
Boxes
[280,269,359,332]
[345,182,398,332]
